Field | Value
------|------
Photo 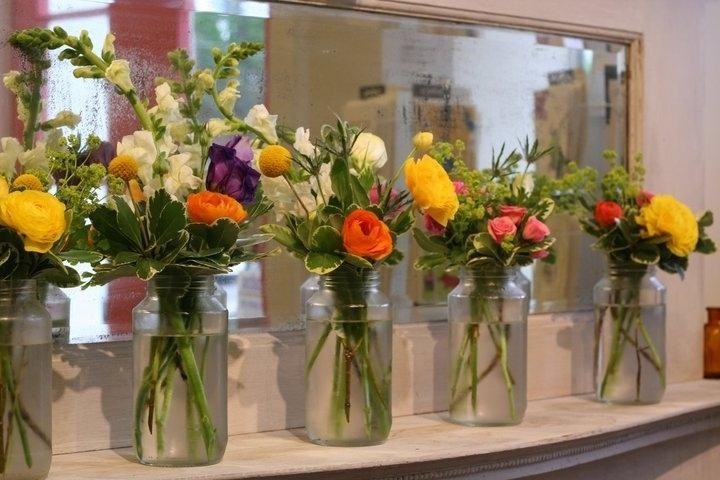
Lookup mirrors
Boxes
[0,0,646,343]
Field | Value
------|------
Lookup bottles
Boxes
[701,305,720,379]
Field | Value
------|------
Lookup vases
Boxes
[587,282,666,407]
[443,281,535,428]
[128,283,232,470]
[303,284,396,446]
[2,283,57,479]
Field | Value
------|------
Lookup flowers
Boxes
[3,28,106,277]
[216,36,458,279]
[15,27,275,279]
[411,126,560,279]
[550,142,714,279]
